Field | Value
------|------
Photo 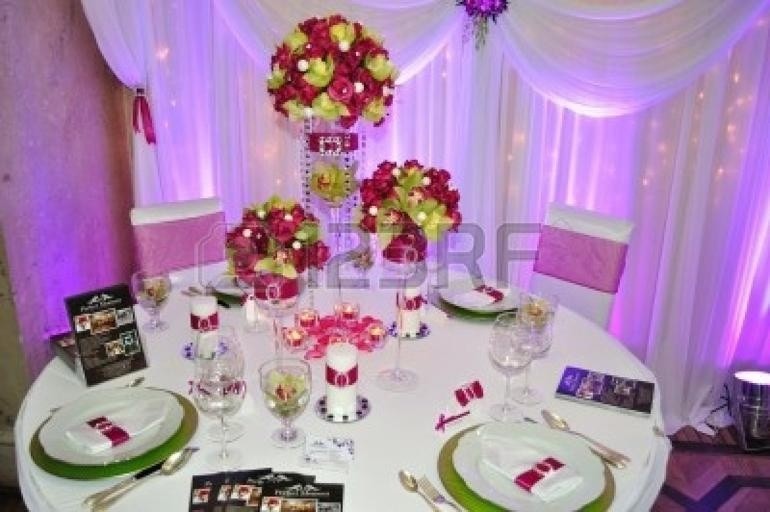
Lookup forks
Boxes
[417,473,463,512]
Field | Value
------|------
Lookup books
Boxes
[63,282,148,387]
[49,330,86,383]
[552,363,656,419]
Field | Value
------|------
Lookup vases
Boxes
[383,214,427,264]
[253,272,298,308]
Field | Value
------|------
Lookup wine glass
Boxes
[131,266,172,335]
[484,286,561,422]
[191,324,246,441]
[257,356,312,450]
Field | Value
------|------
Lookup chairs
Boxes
[128,197,228,298]
[528,202,637,329]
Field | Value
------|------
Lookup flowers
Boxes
[266,13,398,130]
[220,193,331,282]
[353,158,462,253]
[454,1,510,49]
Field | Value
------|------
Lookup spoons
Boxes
[398,469,442,512]
[540,408,631,469]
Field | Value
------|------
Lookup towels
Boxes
[65,400,165,454]
[487,440,584,504]
[454,281,509,309]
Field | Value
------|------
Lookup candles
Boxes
[342,305,354,319]
[323,344,360,417]
[397,284,423,333]
[300,313,314,327]
[285,330,301,347]
[189,296,219,353]
[370,325,385,342]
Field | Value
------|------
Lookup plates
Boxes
[450,419,607,512]
[437,277,520,315]
[37,385,185,468]
